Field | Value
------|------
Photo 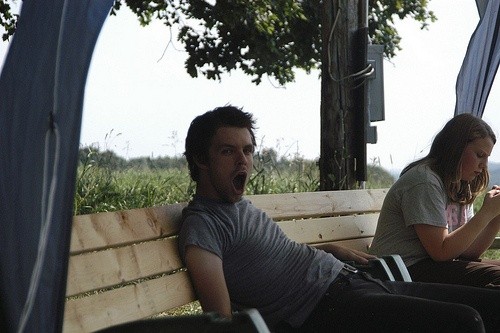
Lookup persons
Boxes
[179,103,500,333]
[367,111,500,290]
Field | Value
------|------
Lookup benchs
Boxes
[62,188,390,332]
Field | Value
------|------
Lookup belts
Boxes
[326,263,357,296]
